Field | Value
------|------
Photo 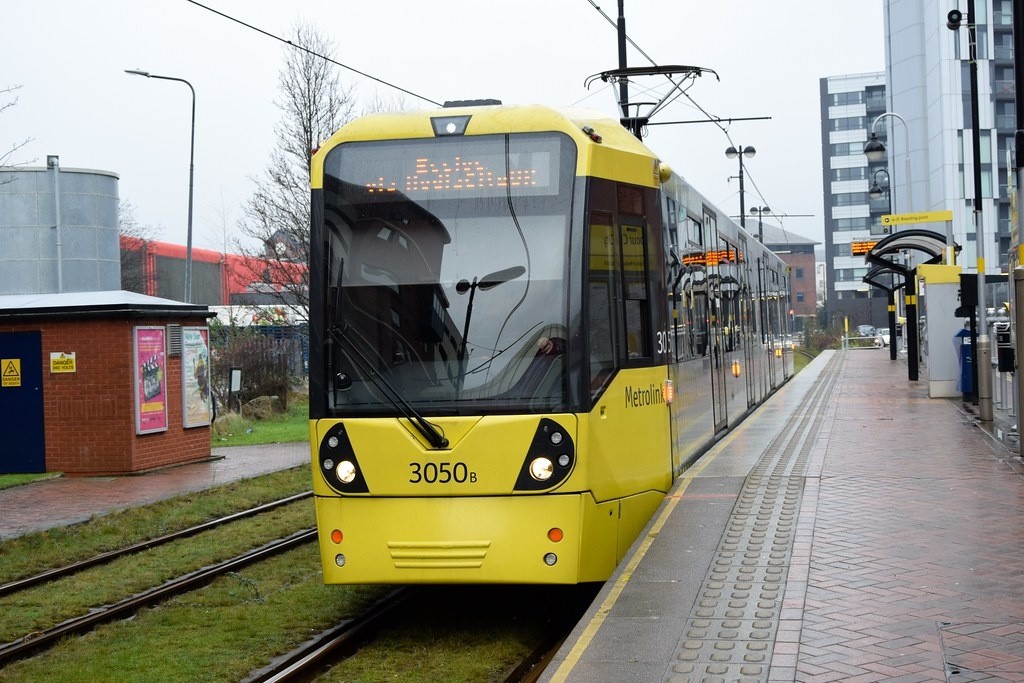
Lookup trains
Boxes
[302,102,798,600]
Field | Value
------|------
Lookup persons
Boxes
[536,335,567,355]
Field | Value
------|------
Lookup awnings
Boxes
[863,228,963,293]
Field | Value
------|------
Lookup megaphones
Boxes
[948,10,962,23]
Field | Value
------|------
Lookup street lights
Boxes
[745,203,770,246]
[862,111,915,215]
[718,134,753,234]
[120,65,198,306]
